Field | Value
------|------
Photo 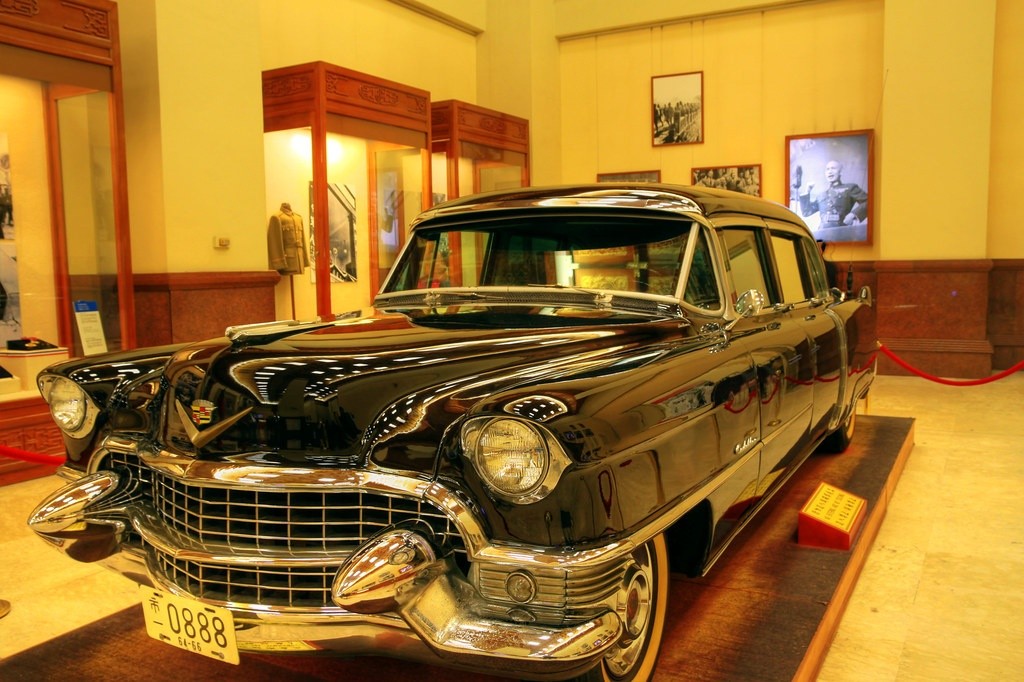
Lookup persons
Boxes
[799,160,869,229]
[692,168,759,196]
[654,101,701,135]
[268,202,310,276]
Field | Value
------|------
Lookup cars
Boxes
[25,180,881,682]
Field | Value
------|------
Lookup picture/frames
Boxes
[784,128,874,247]
[691,164,763,197]
[650,70,705,147]
[596,170,662,185]
